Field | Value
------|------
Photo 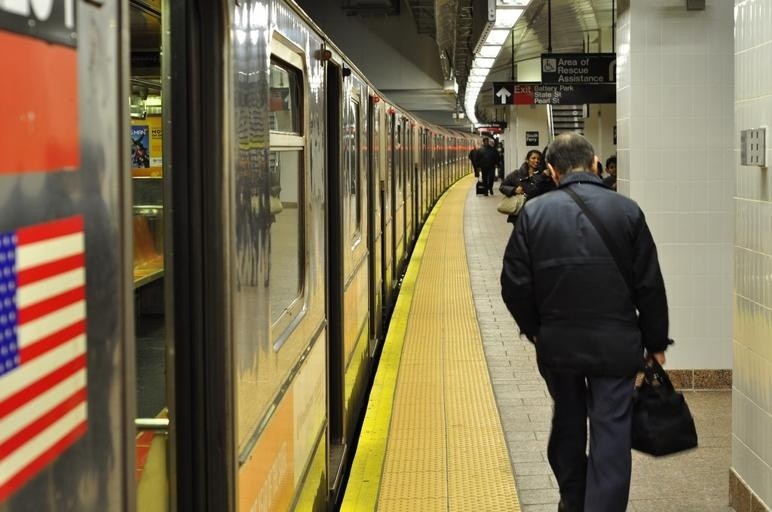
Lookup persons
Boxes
[236,153,280,290]
[499,131,669,512]
[469,137,616,228]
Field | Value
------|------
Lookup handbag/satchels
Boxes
[497,193,527,216]
[630,358,699,459]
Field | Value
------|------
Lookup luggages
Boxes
[475,173,485,195]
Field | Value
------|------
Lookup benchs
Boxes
[133,215,164,329]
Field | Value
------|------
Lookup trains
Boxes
[0,0,491,512]
